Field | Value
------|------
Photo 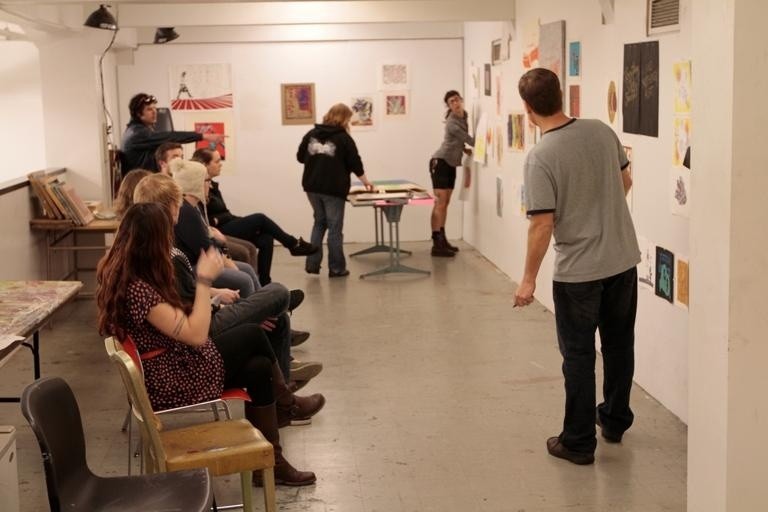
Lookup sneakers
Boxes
[291,237,318,256]
[290,362,323,392]
[290,330,309,347]
[287,289,304,311]
[307,267,349,276]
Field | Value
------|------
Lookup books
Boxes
[28,170,105,227]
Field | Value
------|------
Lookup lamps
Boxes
[152,27,179,45]
[83,0,119,32]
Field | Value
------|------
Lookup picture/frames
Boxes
[280,81,317,127]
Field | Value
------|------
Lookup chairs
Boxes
[102,336,278,511]
[19,375,220,512]
[121,334,253,458]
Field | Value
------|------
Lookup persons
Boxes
[513,67,641,467]
[427,89,475,256]
[115,93,231,179]
[296,102,373,279]
[177,72,192,99]
[95,140,326,488]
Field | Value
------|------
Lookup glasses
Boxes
[138,95,156,106]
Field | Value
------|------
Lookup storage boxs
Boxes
[0,422,20,512]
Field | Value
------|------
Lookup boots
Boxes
[245,397,316,486]
[432,227,458,256]
[271,361,325,429]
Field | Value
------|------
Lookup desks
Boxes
[30,204,125,300]
[0,279,84,404]
[346,179,440,280]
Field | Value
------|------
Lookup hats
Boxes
[170,157,214,238]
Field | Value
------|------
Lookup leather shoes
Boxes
[547,436,594,464]
[595,408,622,443]
